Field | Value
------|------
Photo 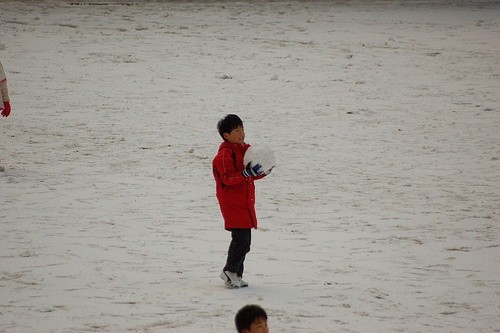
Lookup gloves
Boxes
[263,166,275,177]
[242,162,263,178]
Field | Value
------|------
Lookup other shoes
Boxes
[219,270,249,289]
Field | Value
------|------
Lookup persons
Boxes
[212,113,275,290]
[0,60,12,172]
[234,304,270,333]
